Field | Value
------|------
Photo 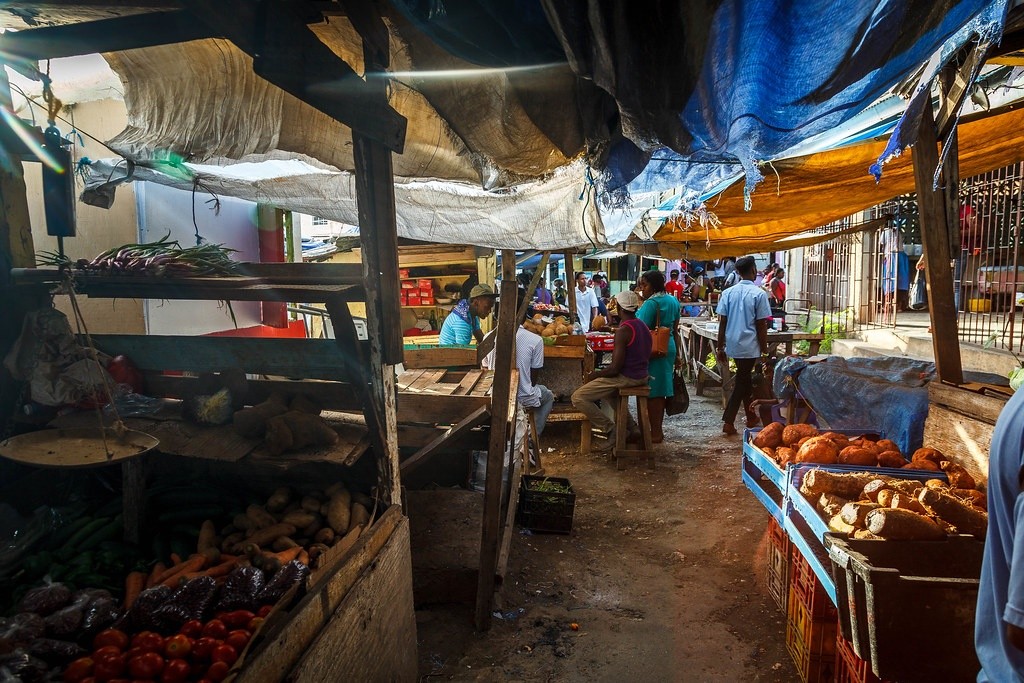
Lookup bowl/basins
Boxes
[969,299,991,312]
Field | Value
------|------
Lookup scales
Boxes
[0,126,160,472]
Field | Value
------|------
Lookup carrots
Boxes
[123,543,326,610]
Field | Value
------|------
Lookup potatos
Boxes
[592,313,606,329]
[752,420,987,501]
[197,479,370,553]
[232,393,340,450]
[523,313,574,338]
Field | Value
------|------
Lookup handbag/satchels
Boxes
[666,367,689,416]
[648,298,670,356]
[908,267,927,310]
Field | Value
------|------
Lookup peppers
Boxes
[0,546,160,617]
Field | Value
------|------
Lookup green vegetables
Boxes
[527,476,574,517]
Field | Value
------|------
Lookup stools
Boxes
[611,386,655,470]
[524,409,545,475]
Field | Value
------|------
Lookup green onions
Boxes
[30,235,244,280]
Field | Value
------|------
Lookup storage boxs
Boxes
[519,474,575,535]
[767,512,984,683]
[589,336,615,350]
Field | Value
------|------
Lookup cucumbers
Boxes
[43,483,248,553]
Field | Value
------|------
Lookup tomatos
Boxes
[61,603,274,683]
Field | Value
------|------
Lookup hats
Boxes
[694,267,704,274]
[614,290,639,311]
[470,284,500,297]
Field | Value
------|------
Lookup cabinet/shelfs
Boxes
[399,248,496,346]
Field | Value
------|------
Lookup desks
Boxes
[692,323,825,409]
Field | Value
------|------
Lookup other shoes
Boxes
[602,427,630,450]
[746,417,759,427]
[627,425,641,441]
[520,441,535,466]
[598,365,606,369]
[723,423,737,434]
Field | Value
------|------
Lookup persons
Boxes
[880,201,988,333]
[480,304,554,467]
[439,284,500,345]
[974,381,1024,683]
[518,256,786,451]
